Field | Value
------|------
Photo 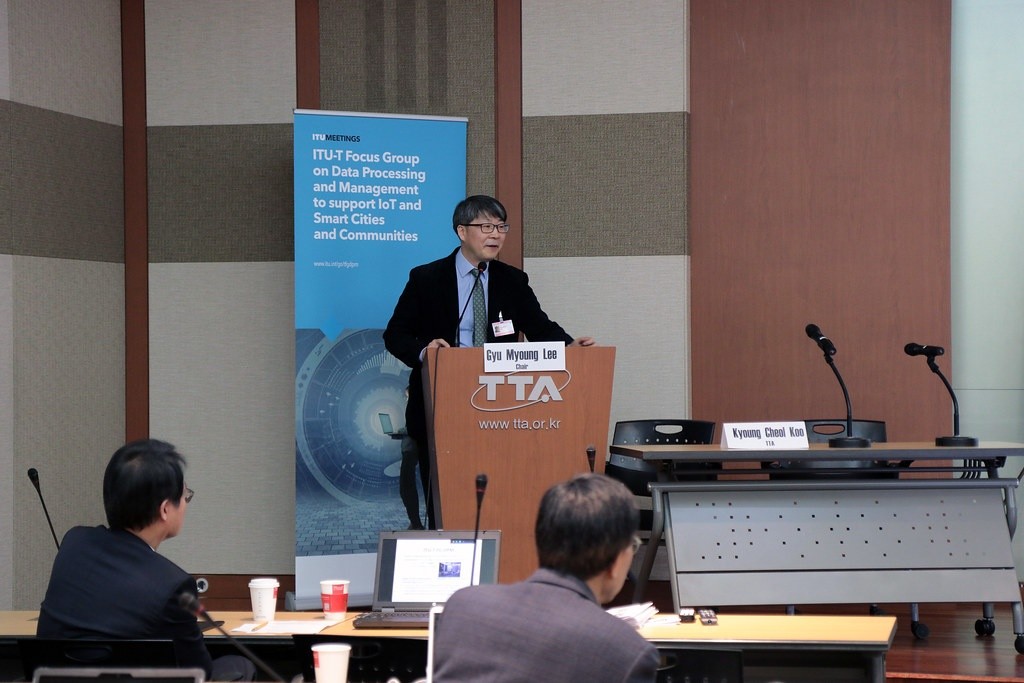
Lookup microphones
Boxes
[586,447,596,472]
[904,343,945,356]
[27,468,60,551]
[806,324,837,355]
[178,592,284,683]
[469,474,487,584]
[455,261,487,348]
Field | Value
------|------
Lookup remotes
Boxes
[699,609,717,625]
[679,608,695,621]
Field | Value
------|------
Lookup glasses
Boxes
[630,533,642,554]
[461,222,510,234]
[181,486,194,502]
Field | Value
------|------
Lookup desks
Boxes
[610,442,1024,653]
[0,613,898,683]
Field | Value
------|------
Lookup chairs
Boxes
[609,419,717,482]
[805,419,899,481]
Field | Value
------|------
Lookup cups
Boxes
[248,578,279,622]
[311,643,352,683]
[320,579,350,621]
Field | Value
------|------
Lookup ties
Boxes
[470,267,487,344]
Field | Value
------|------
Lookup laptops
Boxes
[353,529,502,628]
[32,667,205,683]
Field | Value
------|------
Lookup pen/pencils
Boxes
[252,622,268,631]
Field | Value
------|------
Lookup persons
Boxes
[383,194,601,531]
[36,436,259,683]
[430,473,665,683]
